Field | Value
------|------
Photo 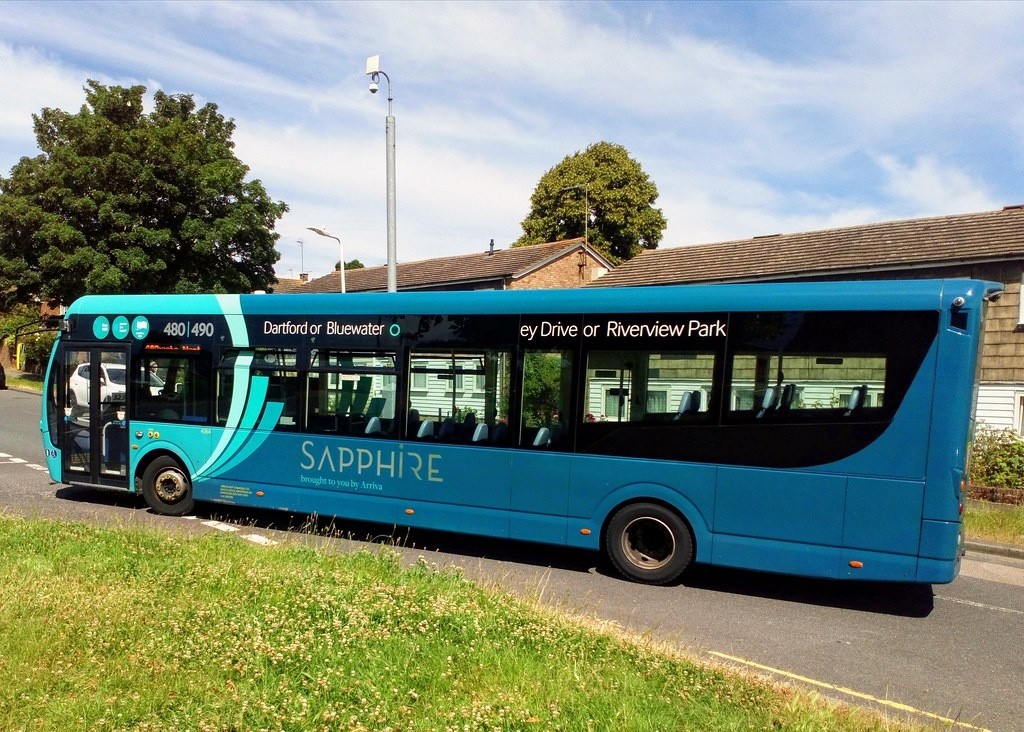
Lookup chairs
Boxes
[490,421,508,443]
[674,390,701,421]
[844,385,867,417]
[774,384,797,420]
[462,412,476,430]
[418,420,435,440]
[409,409,420,432]
[756,386,780,420]
[549,425,561,449]
[441,417,454,439]
[366,417,382,434]
[473,424,489,442]
[533,427,549,449]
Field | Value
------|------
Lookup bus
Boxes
[12,276,1006,593]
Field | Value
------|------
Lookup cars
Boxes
[66,361,182,421]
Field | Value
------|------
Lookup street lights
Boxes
[365,54,399,293]
[305,226,347,295]
